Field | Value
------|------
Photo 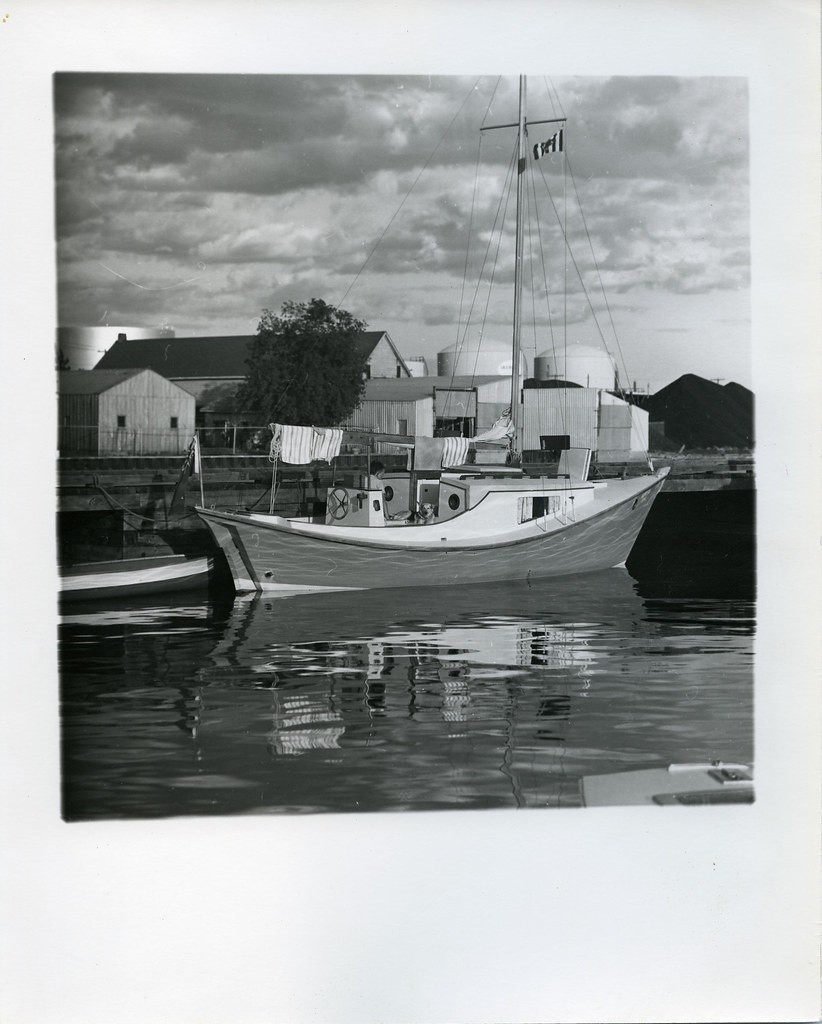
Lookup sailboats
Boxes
[194,76,675,589]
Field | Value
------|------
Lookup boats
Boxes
[61,543,221,602]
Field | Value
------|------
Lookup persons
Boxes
[364,458,401,522]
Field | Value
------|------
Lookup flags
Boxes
[533,127,563,159]
